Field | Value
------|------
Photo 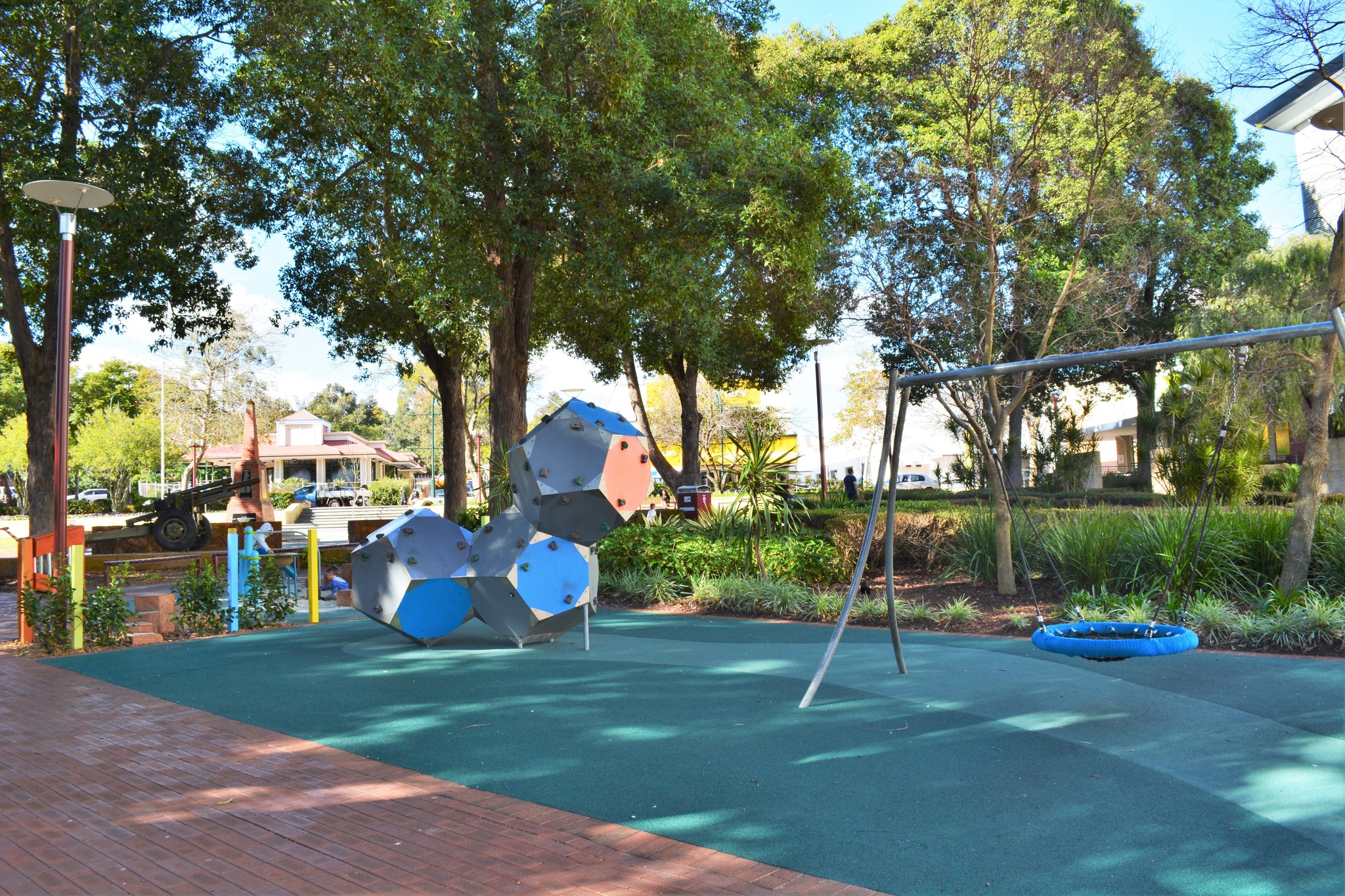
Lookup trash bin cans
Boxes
[18,525,86,643]
[677,485,712,525]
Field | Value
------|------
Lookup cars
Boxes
[429,489,445,497]
[155,468,168,477]
[0,486,19,501]
[67,489,108,507]
[436,473,444,480]
[329,487,356,499]
[466,480,473,496]
[1022,469,1052,486]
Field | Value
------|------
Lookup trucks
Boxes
[289,484,372,507]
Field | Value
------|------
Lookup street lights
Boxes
[698,387,749,482]
[471,430,486,508]
[406,387,443,497]
[187,439,202,487]
[884,473,941,491]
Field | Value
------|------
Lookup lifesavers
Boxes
[965,345,1249,663]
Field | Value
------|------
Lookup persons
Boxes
[661,490,670,509]
[319,566,350,601]
[843,468,860,500]
[408,494,413,501]
[253,522,273,555]
[772,475,793,509]
[412,488,419,498]
[645,503,656,528]
[400,486,408,505]
